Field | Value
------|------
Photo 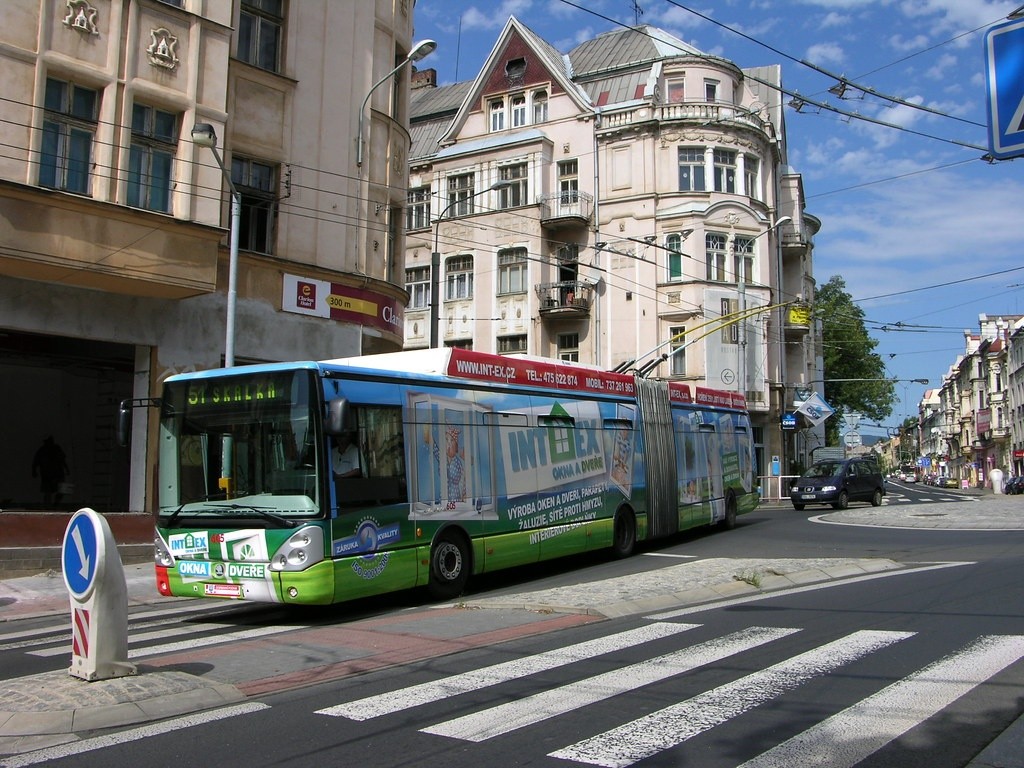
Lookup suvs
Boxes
[790,455,886,512]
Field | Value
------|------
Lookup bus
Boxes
[116,346,758,612]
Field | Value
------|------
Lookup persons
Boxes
[331,433,361,478]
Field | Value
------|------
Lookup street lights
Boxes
[190,117,241,499]
[431,181,513,347]
[739,216,793,394]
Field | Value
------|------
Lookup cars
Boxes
[1010,475,1024,495]
[1004,477,1017,494]
[885,460,959,489]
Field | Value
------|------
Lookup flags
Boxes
[798,392,835,427]
